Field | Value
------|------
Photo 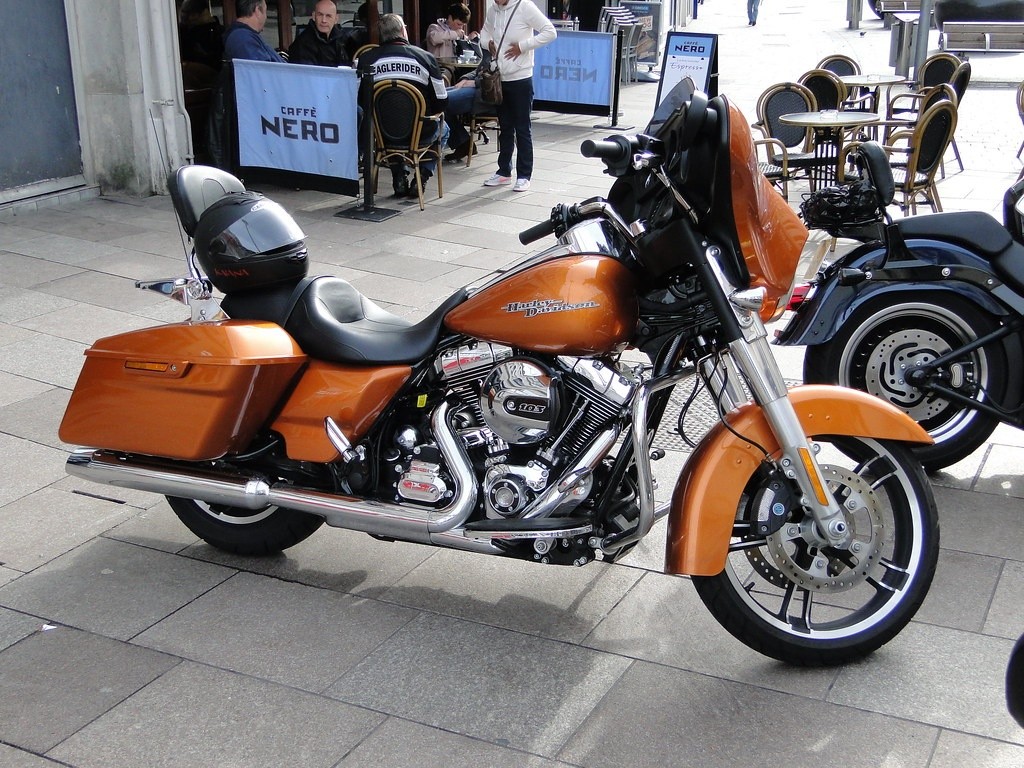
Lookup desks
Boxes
[779,112,880,192]
[437,57,482,85]
[840,75,906,144]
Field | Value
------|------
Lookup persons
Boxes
[747,0,764,26]
[426,4,481,88]
[436,48,492,162]
[358,14,450,197]
[179,0,364,191]
[478,0,558,193]
[698,0,705,5]
[359,0,380,26]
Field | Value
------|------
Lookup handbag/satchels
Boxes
[479,68,503,105]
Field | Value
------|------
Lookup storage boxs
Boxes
[58,320,307,462]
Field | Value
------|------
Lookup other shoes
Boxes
[749,21,756,26]
[409,173,430,199]
[445,141,478,160]
[358,156,364,172]
[392,174,409,197]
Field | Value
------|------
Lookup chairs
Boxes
[181,40,500,210]
[751,54,971,252]
[342,12,365,27]
[597,6,644,86]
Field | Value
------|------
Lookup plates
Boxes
[460,55,477,59]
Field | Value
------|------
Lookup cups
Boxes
[463,50,474,56]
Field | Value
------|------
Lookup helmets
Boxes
[194,190,309,295]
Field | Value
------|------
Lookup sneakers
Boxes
[483,174,512,186]
[512,178,530,190]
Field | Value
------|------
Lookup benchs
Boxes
[934,22,1024,62]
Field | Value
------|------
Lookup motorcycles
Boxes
[770,82,1024,476]
[58,77,942,669]
